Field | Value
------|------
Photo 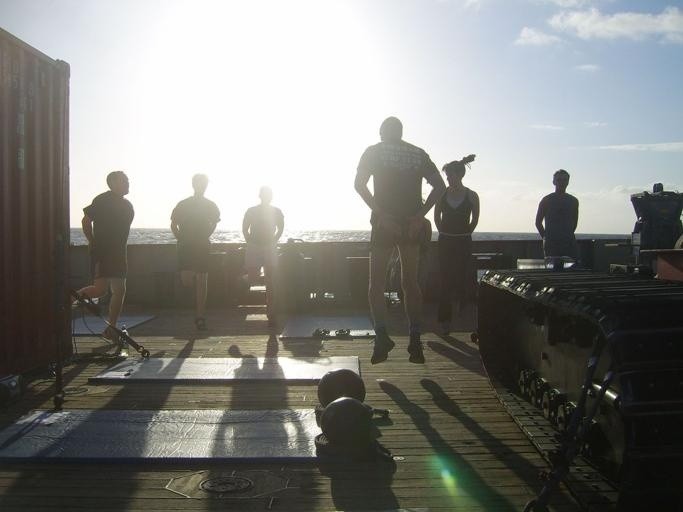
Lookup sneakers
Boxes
[194,318,207,332]
[103,329,120,344]
[407,342,424,364]
[368,335,395,365]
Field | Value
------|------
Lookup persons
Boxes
[71,170,135,335]
[396,218,432,326]
[353,116,447,365]
[240,183,286,337]
[535,170,579,256]
[633,183,663,234]
[433,155,480,330]
[170,173,222,333]
